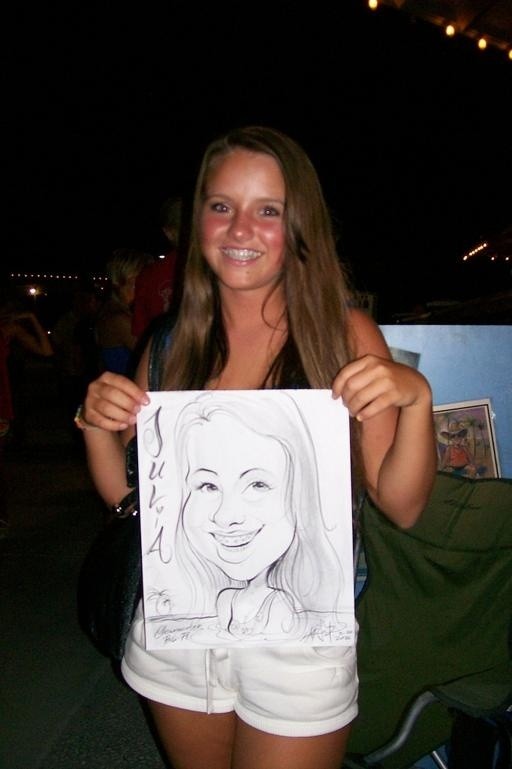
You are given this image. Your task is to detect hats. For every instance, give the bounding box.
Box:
[440,422,468,440]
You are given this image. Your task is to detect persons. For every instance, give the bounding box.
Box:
[441,423,477,479]
[150,392,345,638]
[76,127,439,769]
[3,194,191,445]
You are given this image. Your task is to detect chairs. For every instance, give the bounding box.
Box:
[341,470,512,769]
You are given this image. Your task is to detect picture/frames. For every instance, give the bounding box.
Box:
[432,399,501,480]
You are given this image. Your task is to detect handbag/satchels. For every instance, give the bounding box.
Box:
[75,493,142,685]
